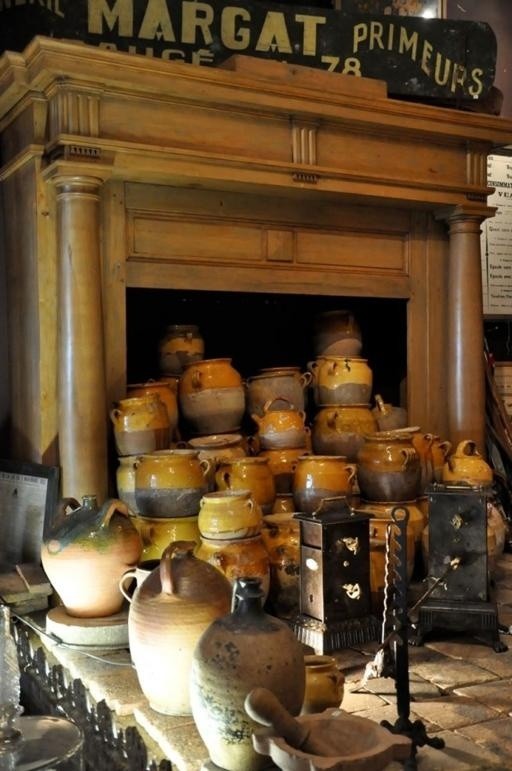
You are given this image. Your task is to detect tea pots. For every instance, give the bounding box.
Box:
[443,440,494,490]
[252,396,306,451]
